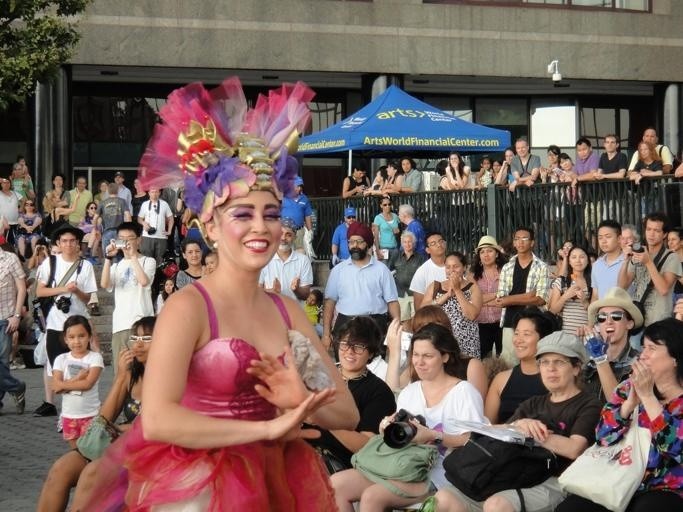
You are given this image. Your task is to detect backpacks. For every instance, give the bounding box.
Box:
[48,253,57,332]
[16,220,41,236]
[442,432,557,501]
[390,270,416,321]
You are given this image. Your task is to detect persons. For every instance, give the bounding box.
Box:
[260,126,682,512]
[116,147,364,512]
[1,157,217,512]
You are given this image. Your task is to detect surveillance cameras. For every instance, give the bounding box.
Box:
[548,61,562,84]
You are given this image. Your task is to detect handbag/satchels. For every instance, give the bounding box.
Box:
[76,414,119,460]
[659,145,681,173]
[350,433,439,498]
[557,404,652,511]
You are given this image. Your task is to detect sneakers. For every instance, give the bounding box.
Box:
[8,359,26,370]
[32,402,57,417]
[10,380,26,414]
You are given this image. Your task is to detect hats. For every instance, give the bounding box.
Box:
[345,222,374,248]
[113,170,124,177]
[293,176,304,186]
[474,234,503,254]
[533,330,587,361]
[51,223,85,246]
[587,287,643,330]
[344,207,356,218]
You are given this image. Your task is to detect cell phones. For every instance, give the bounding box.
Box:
[113,239,128,249]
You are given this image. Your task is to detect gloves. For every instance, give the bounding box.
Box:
[585,334,608,364]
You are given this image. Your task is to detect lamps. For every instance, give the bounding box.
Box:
[548,60,561,81]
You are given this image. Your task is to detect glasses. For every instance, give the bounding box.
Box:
[595,310,629,325]
[336,338,369,354]
[127,335,153,343]
[24,202,35,207]
[89,207,98,210]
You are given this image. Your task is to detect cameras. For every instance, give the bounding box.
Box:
[627,242,644,256]
[148,227,156,235]
[56,296,71,313]
[383,409,429,449]
